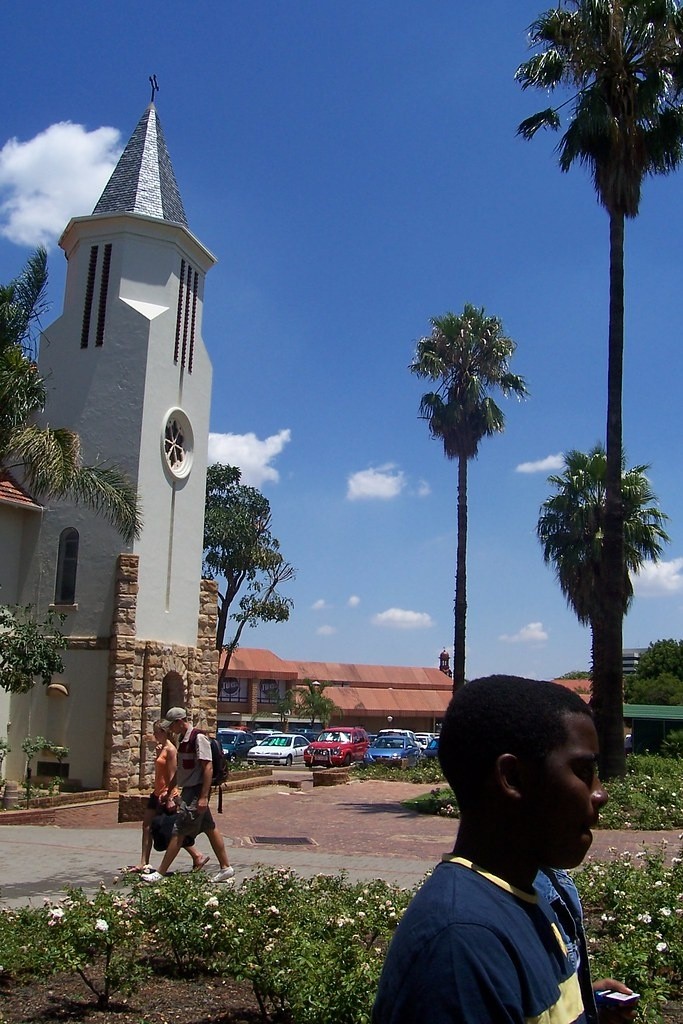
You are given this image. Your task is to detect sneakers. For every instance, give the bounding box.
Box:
[141,871,164,883]
[211,867,235,882]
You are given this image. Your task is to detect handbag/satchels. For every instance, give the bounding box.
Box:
[151,804,194,851]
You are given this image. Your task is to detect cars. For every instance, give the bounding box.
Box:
[230,725,440,759]
[246,733,311,767]
[217,730,259,765]
[363,736,419,767]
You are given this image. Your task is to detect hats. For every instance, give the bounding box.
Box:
[159,707,186,728]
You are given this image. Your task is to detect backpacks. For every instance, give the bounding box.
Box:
[179,729,229,785]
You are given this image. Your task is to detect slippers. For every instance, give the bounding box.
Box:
[192,856,210,873]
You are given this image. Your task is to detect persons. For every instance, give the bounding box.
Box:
[372,674,638,1024]
[139,707,235,884]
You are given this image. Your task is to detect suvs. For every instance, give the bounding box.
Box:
[303,726,371,769]
